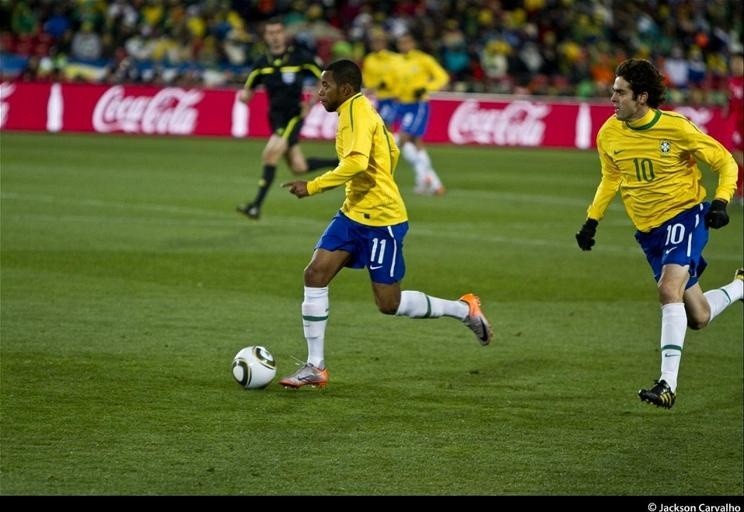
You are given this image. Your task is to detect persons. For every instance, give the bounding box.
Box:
[359,34,451,196]
[575,58,744,409]
[0,0,744,106]
[237,20,341,221]
[279,60,493,388]
[361,36,402,149]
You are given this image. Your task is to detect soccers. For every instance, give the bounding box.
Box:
[232,346,276,389]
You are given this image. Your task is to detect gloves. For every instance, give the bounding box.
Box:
[576,219,598,251]
[705,200,729,229]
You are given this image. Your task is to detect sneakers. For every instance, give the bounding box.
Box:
[279,363,329,388]
[459,293,492,346]
[638,379,676,409]
